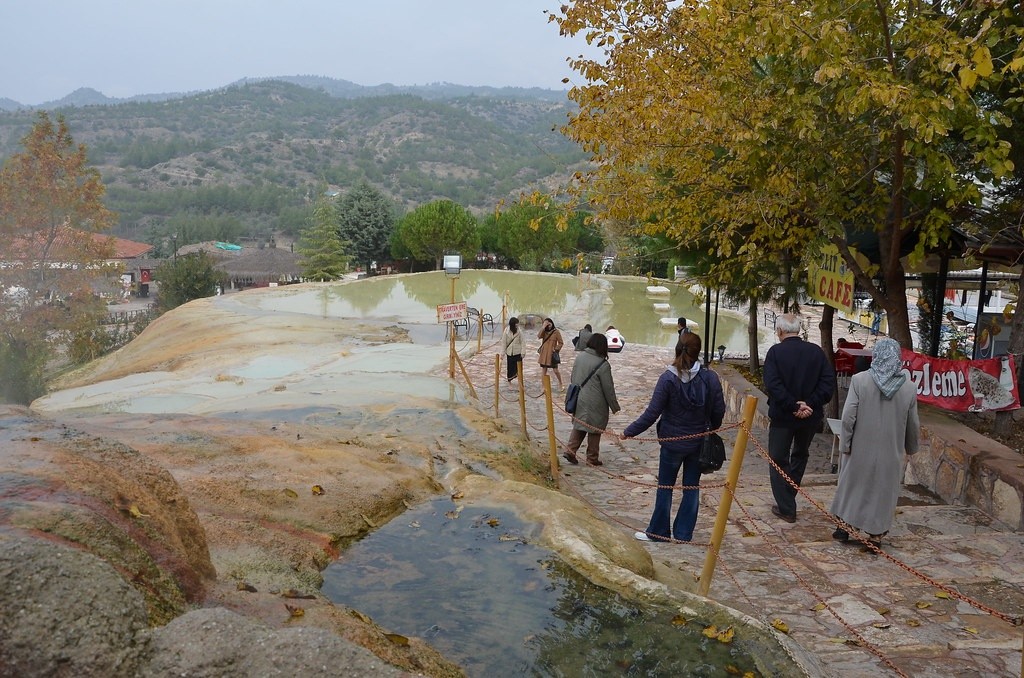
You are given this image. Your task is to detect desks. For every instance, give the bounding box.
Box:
[834,348,872,388]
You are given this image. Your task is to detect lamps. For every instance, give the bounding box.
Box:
[717,345,726,362]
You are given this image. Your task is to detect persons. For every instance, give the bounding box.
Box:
[575,324,593,351]
[563,333,620,466]
[500,317,527,390]
[831,338,919,554]
[619,333,725,542]
[604,325,626,353]
[537,318,564,393]
[677,317,690,341]
[763,314,834,523]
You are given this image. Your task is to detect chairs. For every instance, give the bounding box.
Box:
[444,317,468,341]
[835,357,852,389]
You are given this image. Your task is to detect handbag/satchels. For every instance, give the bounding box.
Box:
[537,349,540,354]
[572,337,580,345]
[698,427,726,474]
[565,384,580,413]
[551,352,560,364]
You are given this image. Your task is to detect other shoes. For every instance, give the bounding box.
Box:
[586,460,602,465]
[563,451,579,464]
[832,528,849,541]
[634,532,653,541]
[866,540,881,555]
[670,532,675,539]
[557,387,564,392]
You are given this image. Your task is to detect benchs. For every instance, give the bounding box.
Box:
[764,308,779,330]
[723,298,739,310]
[465,307,494,339]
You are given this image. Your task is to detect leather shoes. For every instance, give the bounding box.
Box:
[772,506,796,523]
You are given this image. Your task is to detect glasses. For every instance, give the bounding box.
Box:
[774,328,782,335]
